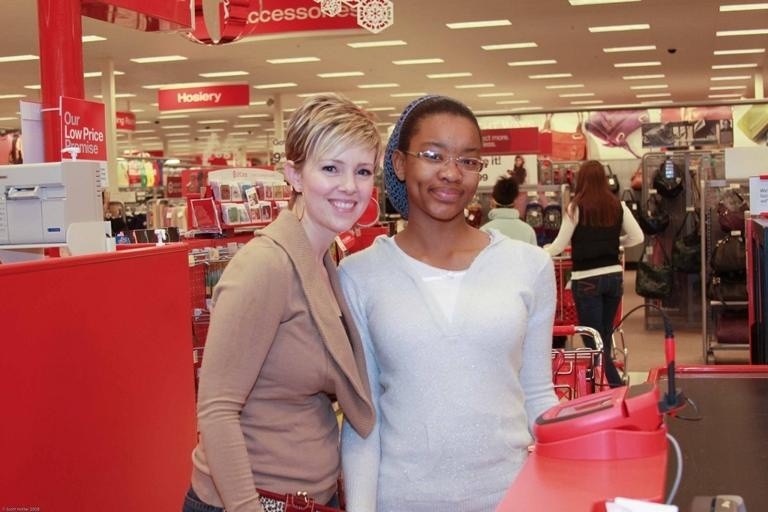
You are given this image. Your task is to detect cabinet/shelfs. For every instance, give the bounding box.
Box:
[107,150,750,366]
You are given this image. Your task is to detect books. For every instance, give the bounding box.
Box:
[210,178,296,225]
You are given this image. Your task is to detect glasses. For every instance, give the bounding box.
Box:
[402,147,486,176]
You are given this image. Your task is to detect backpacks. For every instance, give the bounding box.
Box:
[544,205,562,237]
[524,203,544,233]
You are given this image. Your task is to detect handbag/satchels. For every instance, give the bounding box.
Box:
[652,154,685,198]
[629,161,643,191]
[585,109,652,159]
[671,208,705,274]
[222,487,347,512]
[709,230,758,284]
[634,262,674,299]
[639,196,671,236]
[617,189,641,222]
[540,111,587,161]
[706,276,749,301]
[717,191,750,234]
[601,163,619,194]
[715,309,749,344]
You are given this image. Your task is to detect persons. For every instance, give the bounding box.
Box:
[182,91,383,511]
[476,175,540,246]
[195,92,564,510]
[506,154,527,185]
[543,160,645,389]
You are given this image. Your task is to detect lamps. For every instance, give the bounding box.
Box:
[572,112,583,140]
[540,113,554,134]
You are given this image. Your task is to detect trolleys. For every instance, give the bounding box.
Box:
[552,246,630,401]
